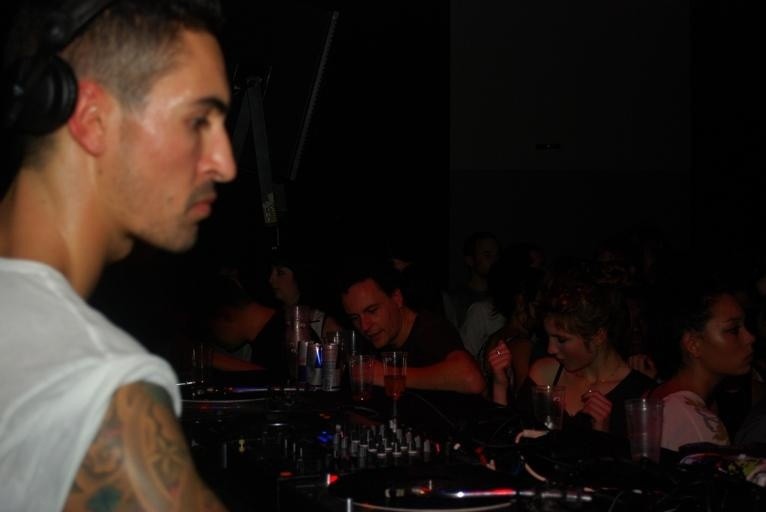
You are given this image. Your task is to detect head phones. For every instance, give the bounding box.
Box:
[0,0,118,137]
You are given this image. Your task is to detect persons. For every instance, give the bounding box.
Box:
[135,263,766,510]
[1,1,237,512]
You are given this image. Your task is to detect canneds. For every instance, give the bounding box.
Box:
[320,343,341,392]
[298,340,314,384]
[305,342,324,388]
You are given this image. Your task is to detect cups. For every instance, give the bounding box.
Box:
[625,397,663,460]
[348,355,375,402]
[531,385,565,431]
[381,351,408,399]
